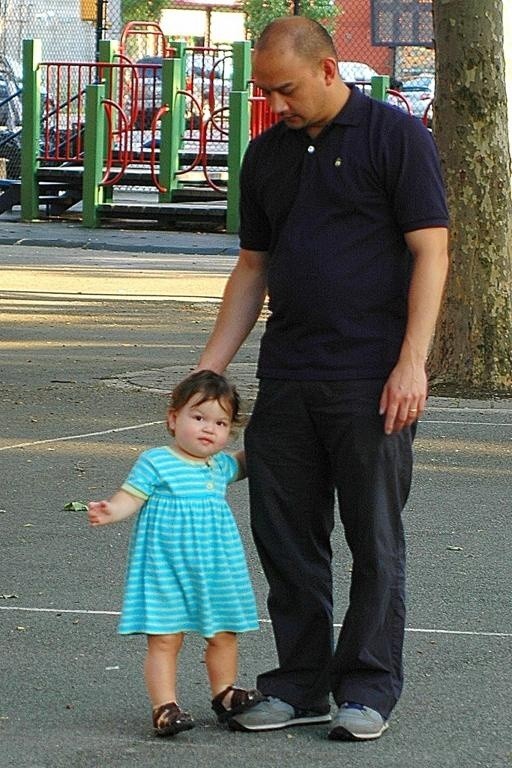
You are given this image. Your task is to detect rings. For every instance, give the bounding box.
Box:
[407,407,418,413]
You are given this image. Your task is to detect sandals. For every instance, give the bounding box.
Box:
[212,686,266,721]
[153,700,195,735]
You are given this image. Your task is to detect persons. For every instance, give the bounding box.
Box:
[166,15,450,741]
[85,368,268,739]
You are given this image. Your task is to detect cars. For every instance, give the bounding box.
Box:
[0,52,61,130]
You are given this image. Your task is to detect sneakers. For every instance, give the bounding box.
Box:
[229,696,332,731]
[329,701,388,740]
